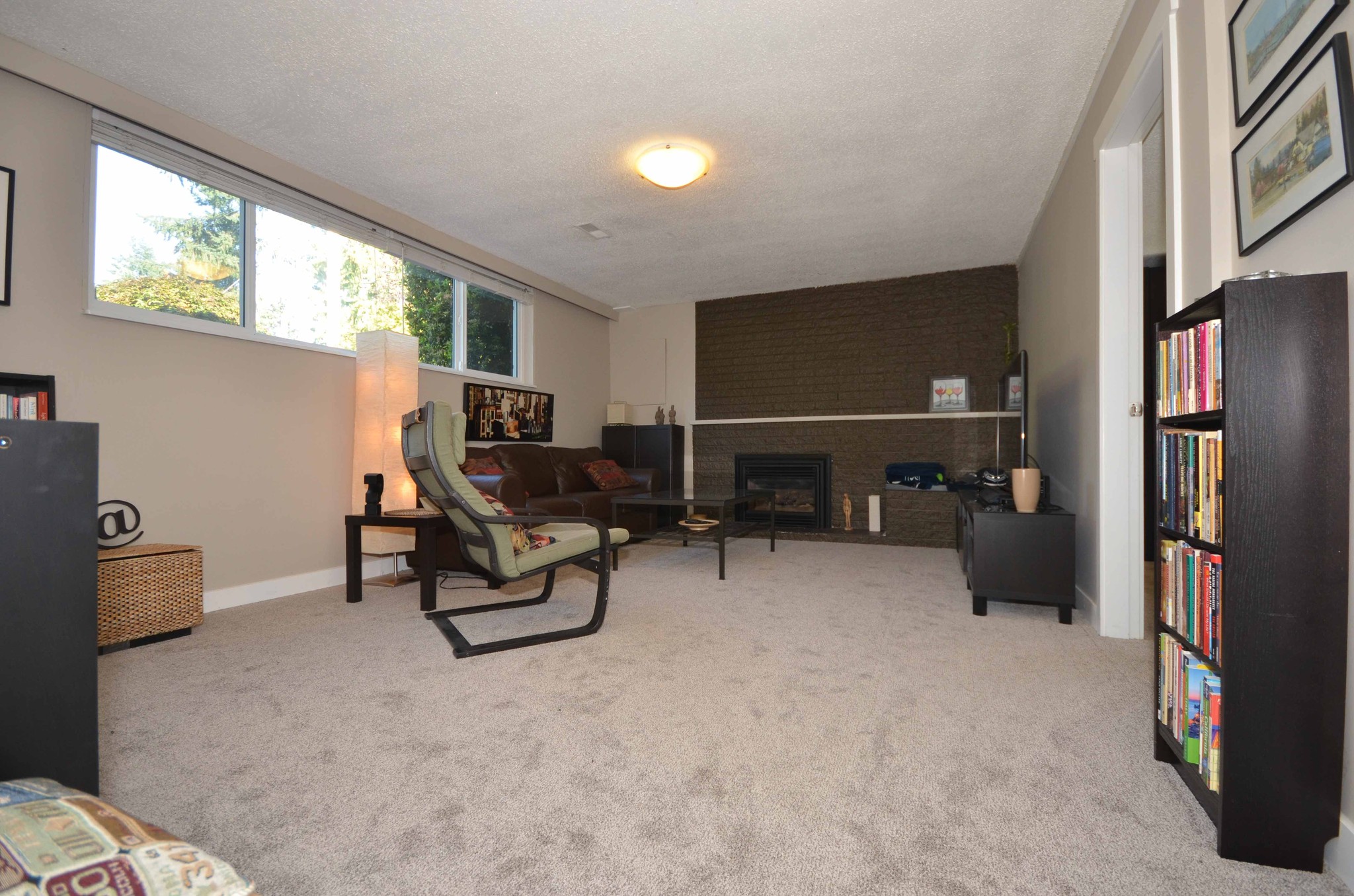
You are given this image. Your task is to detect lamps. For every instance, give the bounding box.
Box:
[352,330,418,588]
[633,144,709,191]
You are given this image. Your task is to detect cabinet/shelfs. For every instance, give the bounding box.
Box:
[955,489,1078,626]
[601,424,685,526]
[1152,272,1348,874]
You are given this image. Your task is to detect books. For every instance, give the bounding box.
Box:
[1159,430,1222,547]
[0,391,48,420]
[1156,319,1222,418]
[1160,540,1222,667]
[1158,633,1221,794]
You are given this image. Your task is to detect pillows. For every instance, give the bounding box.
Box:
[460,458,530,498]
[577,461,638,490]
[0,778,256,896]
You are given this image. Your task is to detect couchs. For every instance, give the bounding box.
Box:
[459,444,661,546]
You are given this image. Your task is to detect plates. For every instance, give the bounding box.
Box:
[679,519,719,530]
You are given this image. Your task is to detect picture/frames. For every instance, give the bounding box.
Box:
[1229,1,1352,125]
[928,374,969,412]
[463,382,554,444]
[0,165,17,308]
[1229,33,1353,256]
[1005,374,1022,411]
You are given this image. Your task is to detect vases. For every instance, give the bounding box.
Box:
[1010,468,1041,512]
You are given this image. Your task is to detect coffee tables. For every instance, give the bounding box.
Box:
[610,490,776,581]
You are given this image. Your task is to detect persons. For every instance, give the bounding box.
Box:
[843,493,852,527]
[669,405,676,424]
[655,406,665,425]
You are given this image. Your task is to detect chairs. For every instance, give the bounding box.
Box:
[399,399,629,659]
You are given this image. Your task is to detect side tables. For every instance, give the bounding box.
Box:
[344,511,455,612]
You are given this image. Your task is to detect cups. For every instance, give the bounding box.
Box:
[1012,468,1040,514]
